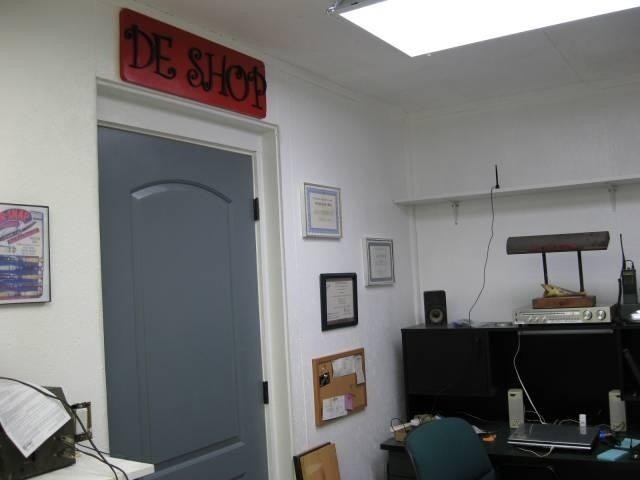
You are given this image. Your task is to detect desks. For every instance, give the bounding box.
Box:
[380,418,640,480]
[27,444,155,480]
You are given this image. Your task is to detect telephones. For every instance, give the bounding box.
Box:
[618,234,640,326]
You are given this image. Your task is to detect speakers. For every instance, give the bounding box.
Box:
[423,289,447,326]
[508,389,526,430]
[608,388,627,433]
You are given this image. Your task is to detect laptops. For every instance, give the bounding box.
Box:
[505,422,599,452]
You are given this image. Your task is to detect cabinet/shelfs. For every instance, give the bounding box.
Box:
[400,319,640,424]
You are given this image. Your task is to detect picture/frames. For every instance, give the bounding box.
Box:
[318,273,358,332]
[362,236,397,287]
[299,180,342,240]
[312,348,367,428]
[0,202,52,306]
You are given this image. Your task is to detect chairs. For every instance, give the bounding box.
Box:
[404,418,496,479]
